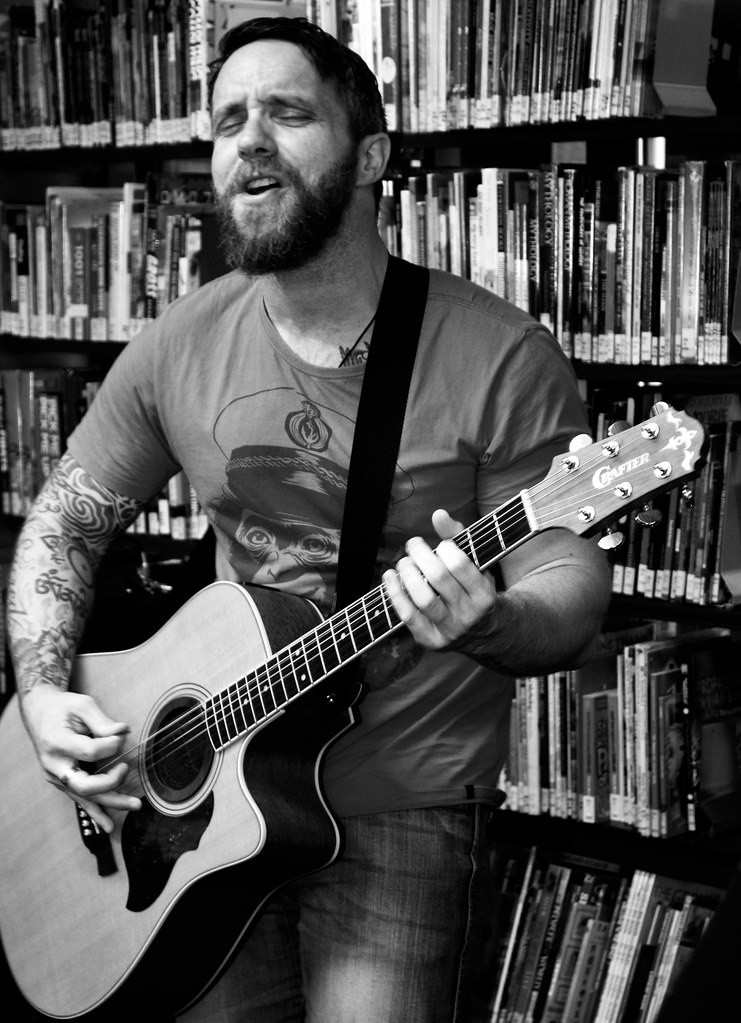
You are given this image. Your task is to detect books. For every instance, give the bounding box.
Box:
[0,1,741,1023]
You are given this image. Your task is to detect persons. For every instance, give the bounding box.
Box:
[6,15,612,1023]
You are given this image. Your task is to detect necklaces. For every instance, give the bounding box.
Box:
[338,315,375,368]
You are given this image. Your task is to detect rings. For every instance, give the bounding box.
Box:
[62,767,81,786]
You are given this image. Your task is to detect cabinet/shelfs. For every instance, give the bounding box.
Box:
[0,1,741,1023]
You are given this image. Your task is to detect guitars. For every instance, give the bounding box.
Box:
[0,396,709,1022]
